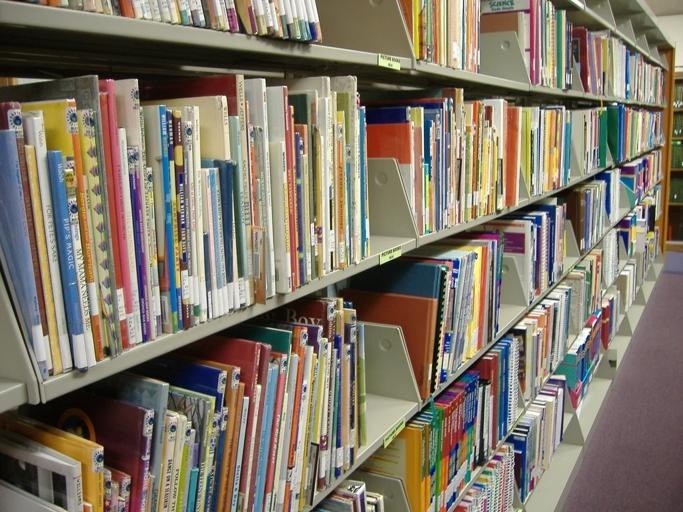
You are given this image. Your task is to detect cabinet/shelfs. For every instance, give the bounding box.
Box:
[663,72,683,253]
[0,0,676,512]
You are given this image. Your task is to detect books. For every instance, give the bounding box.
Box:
[1,2,670,512]
